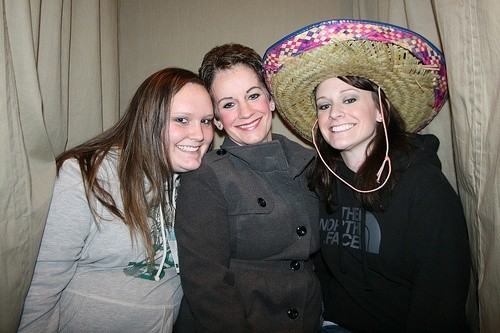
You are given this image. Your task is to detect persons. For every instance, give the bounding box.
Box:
[260,19,472,332]
[169,43,324,333]
[17,66,215,333]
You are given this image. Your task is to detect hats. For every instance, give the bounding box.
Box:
[262,20,449,147]
